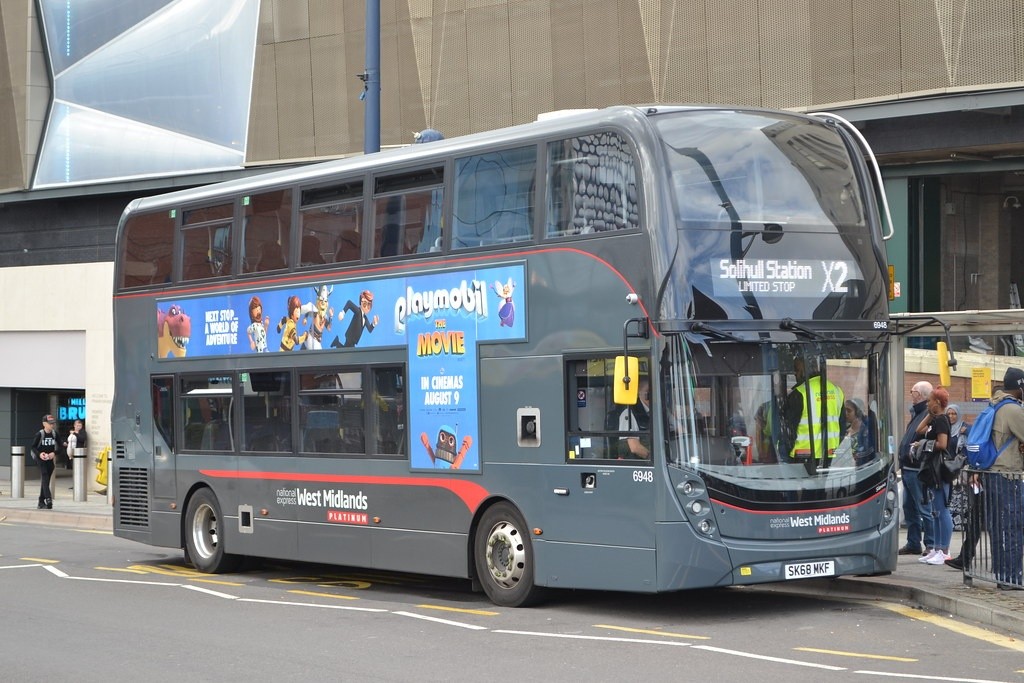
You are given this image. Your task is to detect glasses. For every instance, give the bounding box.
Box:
[909,389,919,394]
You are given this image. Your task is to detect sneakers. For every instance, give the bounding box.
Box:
[926,549,951,565]
[945,553,972,571]
[918,549,939,563]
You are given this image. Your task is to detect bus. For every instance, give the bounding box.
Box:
[110,102,958,610]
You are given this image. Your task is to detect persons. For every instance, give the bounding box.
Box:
[755,354,868,464]
[62,420,86,490]
[617,380,687,459]
[32,415,63,508]
[898,367,1024,590]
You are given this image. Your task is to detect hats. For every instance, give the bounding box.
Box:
[1003,367,1024,390]
[41,414,57,424]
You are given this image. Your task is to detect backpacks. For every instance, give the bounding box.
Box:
[30,429,56,461]
[965,398,1022,470]
[939,448,968,483]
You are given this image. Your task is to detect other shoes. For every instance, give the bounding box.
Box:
[922,547,934,555]
[997,577,1024,590]
[899,541,922,554]
[38,497,52,509]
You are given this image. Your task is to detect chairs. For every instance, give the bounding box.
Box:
[186,411,348,453]
[183,223,442,280]
